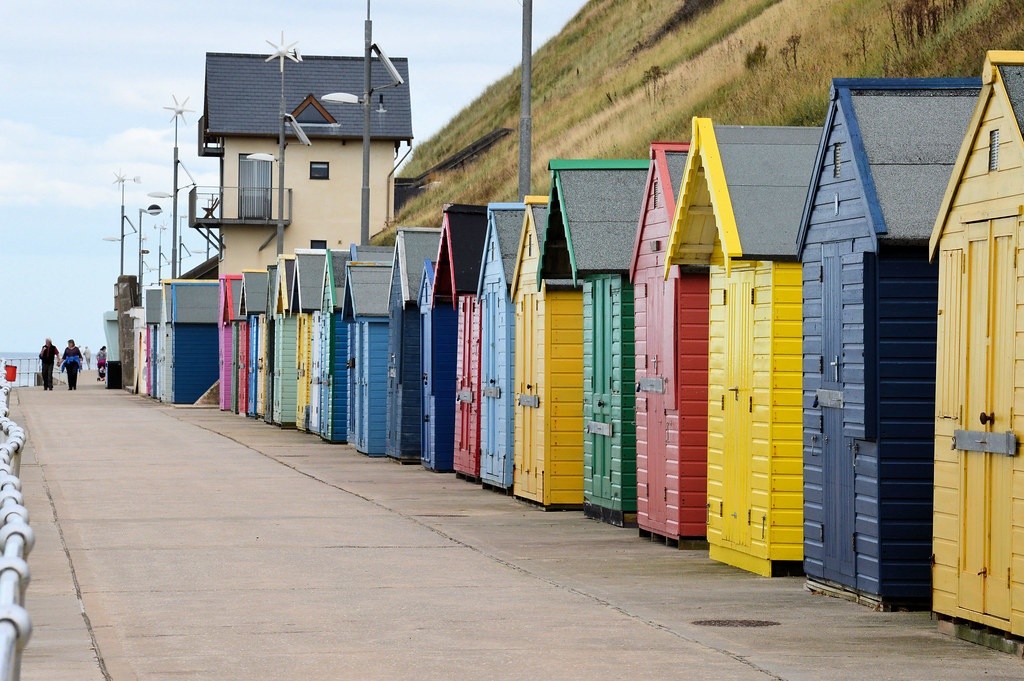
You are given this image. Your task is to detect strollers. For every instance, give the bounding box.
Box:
[98,358,106,381]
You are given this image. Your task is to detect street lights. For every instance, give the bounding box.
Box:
[320,91,370,246]
[138,204,162,308]
[247,153,285,256]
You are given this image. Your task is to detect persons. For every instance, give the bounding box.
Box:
[95,346,106,362]
[38,338,61,392]
[56,340,82,391]
[82,347,91,371]
[98,366,105,381]
